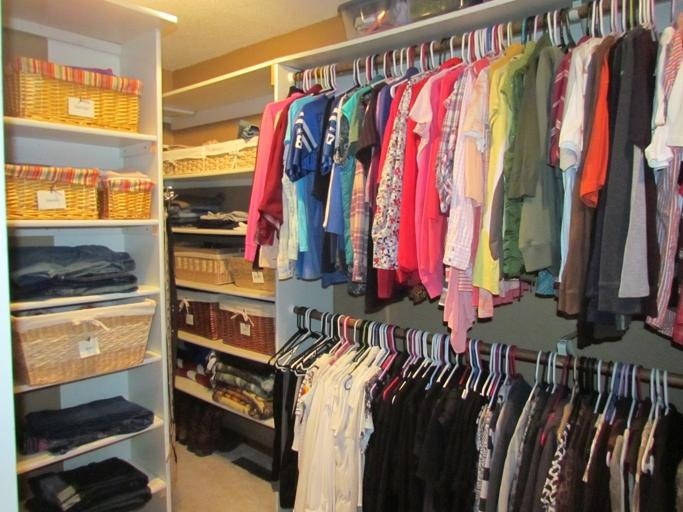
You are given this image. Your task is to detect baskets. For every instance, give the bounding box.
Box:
[173,247,244,285]
[5,163,101,220]
[164,151,174,176]
[204,139,235,173]
[228,256,277,291]
[219,296,276,356]
[97,177,156,220]
[176,289,227,341]
[11,297,156,386]
[235,136,259,169]
[3,56,143,133]
[174,146,205,176]
